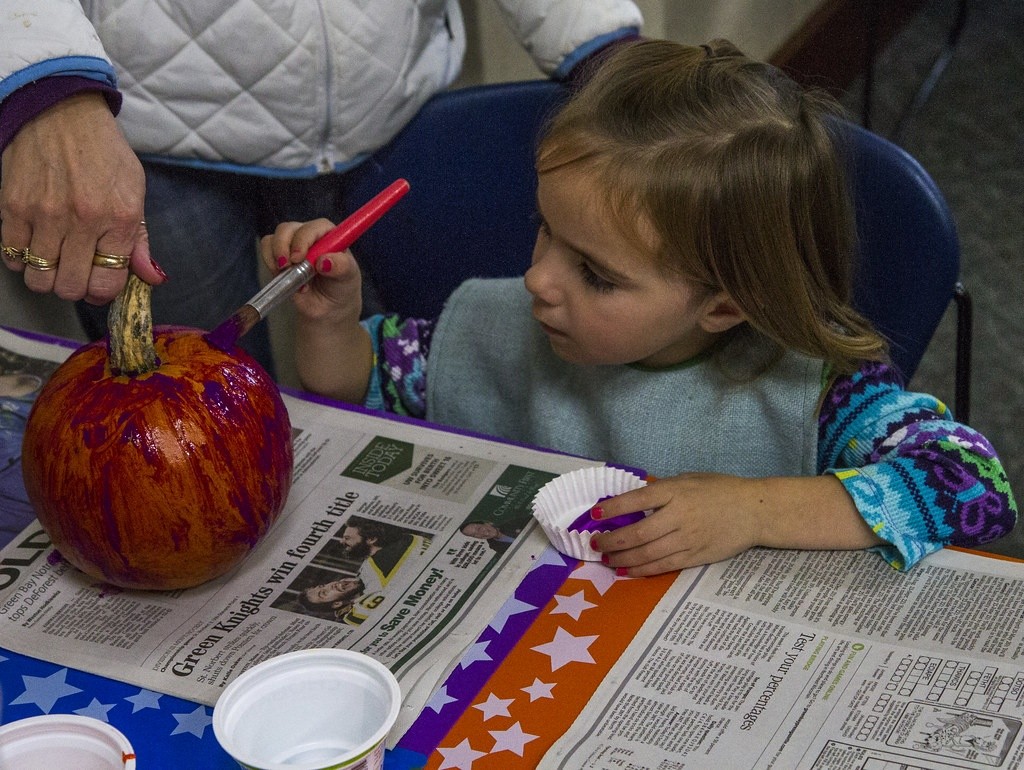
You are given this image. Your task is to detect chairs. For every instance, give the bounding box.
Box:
[350,62,982,422]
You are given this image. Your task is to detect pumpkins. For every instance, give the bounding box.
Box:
[23,269,297,590]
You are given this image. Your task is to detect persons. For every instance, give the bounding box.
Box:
[260,35,1020,580]
[2,0,653,363]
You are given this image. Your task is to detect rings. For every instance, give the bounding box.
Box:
[26,254,60,274]
[91,250,134,270]
[0,242,29,259]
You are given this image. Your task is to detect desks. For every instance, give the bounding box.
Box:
[1,322,1024,769]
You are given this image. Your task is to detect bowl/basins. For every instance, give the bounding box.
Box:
[0,714,136,770]
[211,647,402,770]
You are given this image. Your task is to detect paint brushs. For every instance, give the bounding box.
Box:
[198,176,415,355]
